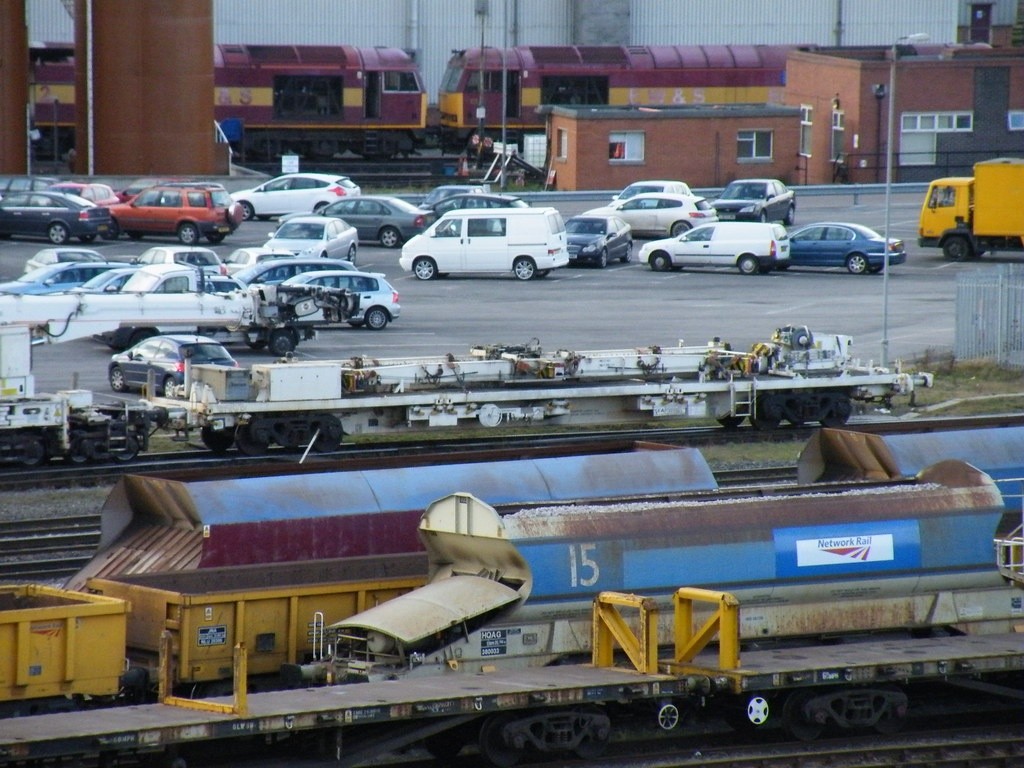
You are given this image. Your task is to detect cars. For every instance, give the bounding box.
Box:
[230,173,361,222]
[710,179,796,227]
[49,183,120,207]
[262,217,357,266]
[0,192,111,244]
[0,248,400,398]
[278,196,436,248]
[416,185,528,218]
[773,222,906,275]
[0,175,57,198]
[565,182,790,275]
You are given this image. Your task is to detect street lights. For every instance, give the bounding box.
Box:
[880,32,929,367]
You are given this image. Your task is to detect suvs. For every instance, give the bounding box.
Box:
[99,182,243,246]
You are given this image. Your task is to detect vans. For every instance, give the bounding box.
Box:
[398,208,571,282]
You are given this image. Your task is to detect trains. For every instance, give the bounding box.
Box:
[62,415,1024,586]
[0,588,1024,768]
[27,42,804,163]
[0,285,935,467]
[279,461,1024,690]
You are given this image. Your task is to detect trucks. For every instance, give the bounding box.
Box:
[918,161,1024,261]
